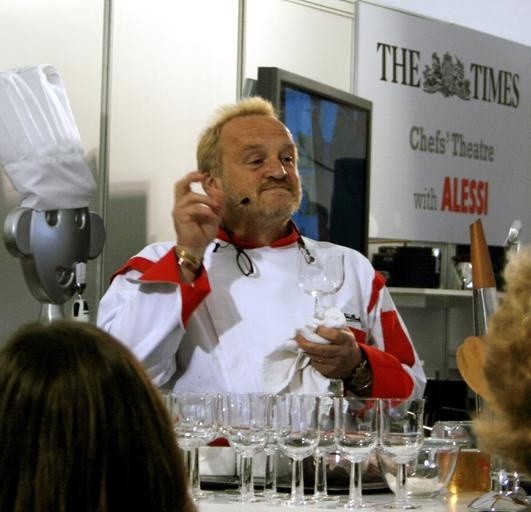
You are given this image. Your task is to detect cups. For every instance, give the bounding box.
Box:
[437,420,492,495]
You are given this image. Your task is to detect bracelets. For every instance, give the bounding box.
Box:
[175,246,202,276]
[343,359,373,396]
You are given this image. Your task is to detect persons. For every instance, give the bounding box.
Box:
[96,98,427,479]
[0,320,197,512]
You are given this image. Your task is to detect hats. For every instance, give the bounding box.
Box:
[0,51,96,210]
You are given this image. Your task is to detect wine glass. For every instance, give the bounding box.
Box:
[158,391,426,512]
[298,250,345,318]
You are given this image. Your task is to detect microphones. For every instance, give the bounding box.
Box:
[217,194,252,210]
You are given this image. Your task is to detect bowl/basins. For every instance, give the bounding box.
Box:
[369,437,467,499]
